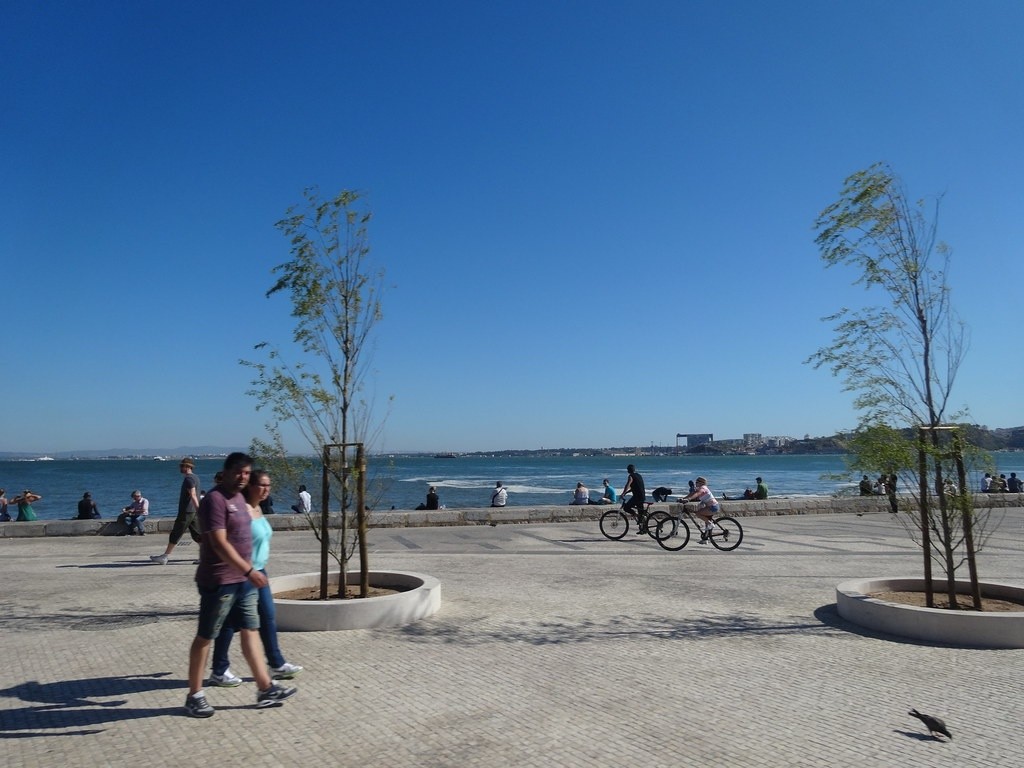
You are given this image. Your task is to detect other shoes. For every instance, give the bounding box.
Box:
[636,530,647,534]
[704,523,716,531]
[697,539,708,545]
[636,514,644,525]
[140,532,145,535]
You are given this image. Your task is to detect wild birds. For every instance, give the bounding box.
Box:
[909,708,953,740]
[365,505,369,510]
[391,505,395,511]
[857,512,864,517]
[489,520,497,527]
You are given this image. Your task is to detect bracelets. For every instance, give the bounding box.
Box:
[243,567,254,576]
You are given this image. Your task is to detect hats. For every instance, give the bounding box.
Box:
[180,458,195,468]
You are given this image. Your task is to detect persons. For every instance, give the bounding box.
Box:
[860,470,899,514]
[291,485,312,513]
[653,487,672,502]
[743,477,768,500]
[185,452,298,718]
[569,482,589,505]
[944,473,1024,497]
[426,486,439,510]
[678,476,719,545]
[150,458,202,565]
[0,488,13,522]
[200,472,276,515]
[78,492,101,519]
[490,481,508,507]
[120,490,150,535]
[598,478,616,504]
[208,469,303,687]
[619,464,647,535]
[9,490,42,521]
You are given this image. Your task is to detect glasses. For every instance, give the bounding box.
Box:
[256,483,272,488]
[696,482,699,483]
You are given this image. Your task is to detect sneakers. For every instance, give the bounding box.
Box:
[150,555,169,565]
[257,679,298,707]
[184,690,215,718]
[208,667,243,687]
[193,559,201,564]
[268,663,304,678]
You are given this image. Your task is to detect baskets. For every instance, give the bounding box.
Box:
[669,503,684,516]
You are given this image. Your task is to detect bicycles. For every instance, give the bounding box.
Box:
[599,494,676,541]
[655,498,743,551]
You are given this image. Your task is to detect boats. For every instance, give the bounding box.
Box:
[153,457,166,461]
[35,455,55,464]
[434,453,456,458]
